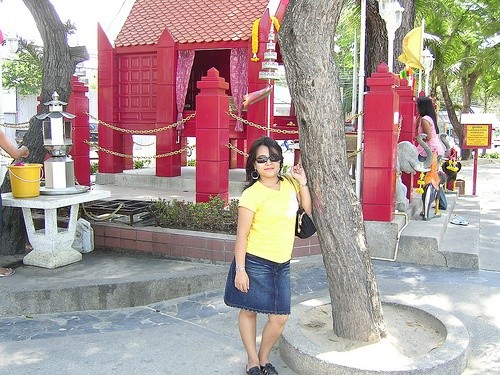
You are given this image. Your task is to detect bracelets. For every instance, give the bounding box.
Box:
[298,183,308,187]
[236,266,245,272]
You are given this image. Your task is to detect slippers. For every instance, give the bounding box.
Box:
[245,365,263,375]
[260,363,278,375]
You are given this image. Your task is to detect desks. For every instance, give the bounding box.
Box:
[1,190,111,268]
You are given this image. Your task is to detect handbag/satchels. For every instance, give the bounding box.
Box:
[281,173,317,239]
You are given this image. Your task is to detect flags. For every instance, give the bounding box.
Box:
[397,19,425,71]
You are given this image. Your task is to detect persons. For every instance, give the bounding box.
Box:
[416,97,447,210]
[224,138,312,375]
[0,131,29,276]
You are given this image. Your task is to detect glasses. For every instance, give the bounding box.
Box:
[254,154,281,163]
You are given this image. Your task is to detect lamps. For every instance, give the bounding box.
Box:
[35,91,90,194]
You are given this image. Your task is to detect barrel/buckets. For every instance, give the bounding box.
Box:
[6,163,43,197]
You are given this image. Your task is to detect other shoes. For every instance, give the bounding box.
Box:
[451,214,469,226]
[0,267,15,277]
